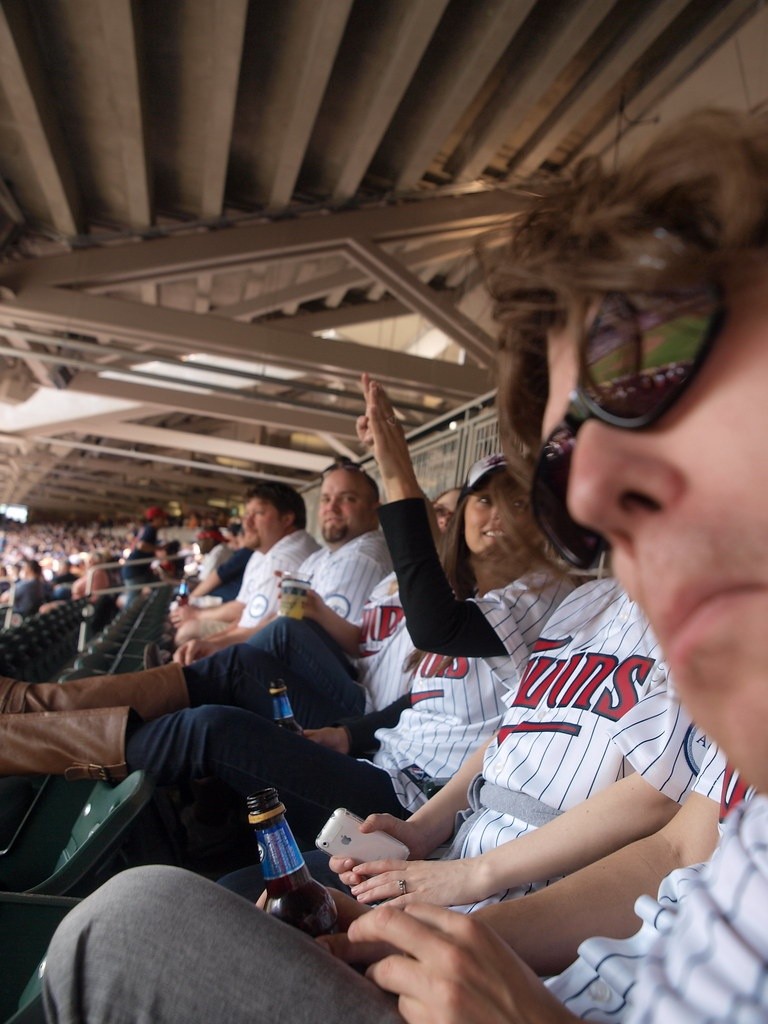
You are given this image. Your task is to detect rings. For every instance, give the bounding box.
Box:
[387,414,397,427]
[398,880,407,896]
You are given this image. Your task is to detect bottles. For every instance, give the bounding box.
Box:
[176,579,188,606]
[246,788,340,938]
[268,679,303,733]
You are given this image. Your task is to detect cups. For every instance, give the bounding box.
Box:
[278,571,312,620]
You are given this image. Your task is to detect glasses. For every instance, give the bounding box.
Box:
[528,228,724,568]
[322,461,376,492]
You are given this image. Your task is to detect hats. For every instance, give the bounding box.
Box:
[455,453,507,512]
[145,507,169,522]
[198,529,231,542]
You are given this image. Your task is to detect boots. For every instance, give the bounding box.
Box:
[0,663,191,720]
[0,704,146,781]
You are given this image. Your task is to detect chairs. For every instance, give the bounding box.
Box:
[0,769,155,895]
[0,586,175,684]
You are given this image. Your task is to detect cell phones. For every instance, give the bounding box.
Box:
[316,807,409,877]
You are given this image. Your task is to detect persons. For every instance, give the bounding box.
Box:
[198,575,718,948]
[489,94,768,1024]
[1,375,588,875]
[38,734,761,1024]
